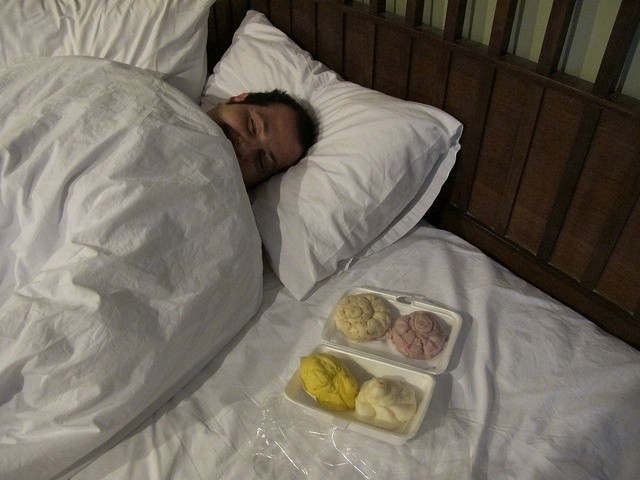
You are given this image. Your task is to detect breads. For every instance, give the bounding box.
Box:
[334,291,391,343]
[355,376,417,427]
[389,310,446,360]
[299,353,357,410]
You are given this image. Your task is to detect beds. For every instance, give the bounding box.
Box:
[0,2,639,479]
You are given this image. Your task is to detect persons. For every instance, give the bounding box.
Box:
[202,88,318,192]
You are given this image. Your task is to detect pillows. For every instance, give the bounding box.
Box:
[1,1,215,104]
[195,7,466,302]
